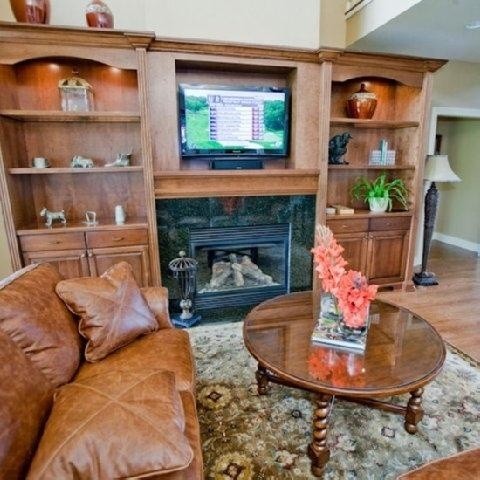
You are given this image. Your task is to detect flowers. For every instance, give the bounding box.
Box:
[311,223,378,329]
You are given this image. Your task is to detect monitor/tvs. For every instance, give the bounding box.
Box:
[177,82,291,170]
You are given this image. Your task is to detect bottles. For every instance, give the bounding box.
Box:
[114,204,126,224]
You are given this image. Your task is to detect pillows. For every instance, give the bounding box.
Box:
[23,369,195,480]
[54,261,159,362]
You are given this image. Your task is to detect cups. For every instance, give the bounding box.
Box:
[31,158,52,169]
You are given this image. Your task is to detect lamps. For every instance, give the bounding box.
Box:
[413,151,461,286]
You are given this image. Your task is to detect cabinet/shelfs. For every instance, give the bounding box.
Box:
[314,44,449,295]
[0,20,164,289]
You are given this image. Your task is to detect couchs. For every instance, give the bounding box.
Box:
[0,258,205,480]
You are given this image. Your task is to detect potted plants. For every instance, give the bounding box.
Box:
[350,170,411,214]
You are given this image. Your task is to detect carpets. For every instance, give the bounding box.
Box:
[171,316,480,480]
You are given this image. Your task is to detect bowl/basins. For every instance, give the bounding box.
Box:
[345,98,378,119]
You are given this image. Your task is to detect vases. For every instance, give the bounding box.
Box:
[335,317,369,341]
[10,0,114,29]
